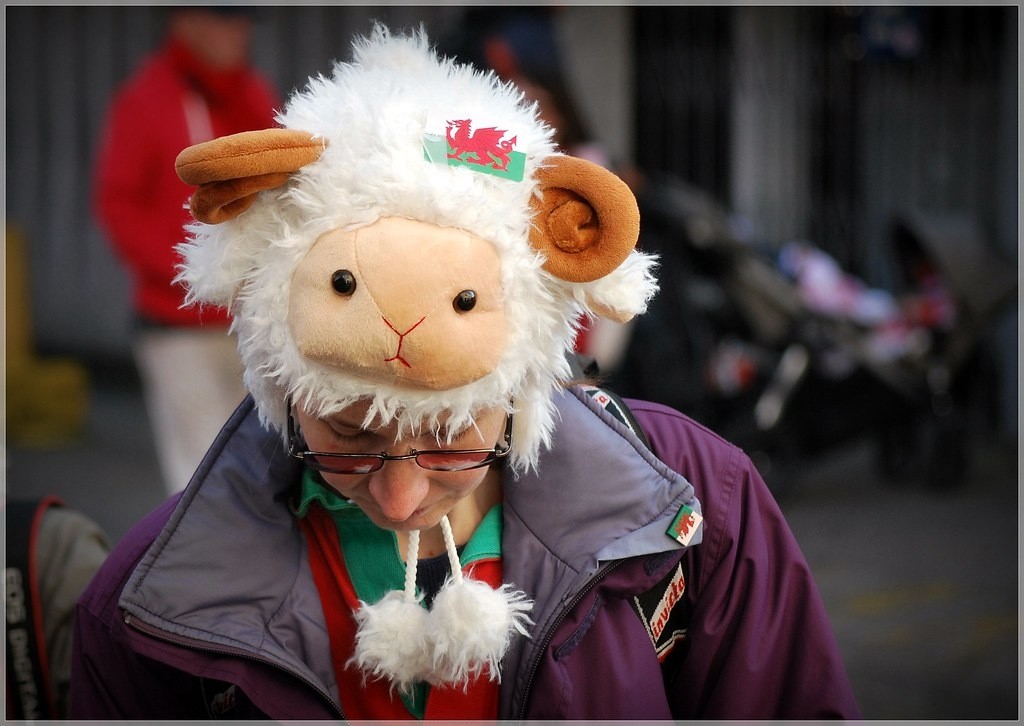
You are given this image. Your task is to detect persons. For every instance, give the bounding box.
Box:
[88,5,969,499]
[63,19,866,721]
[6,492,115,720]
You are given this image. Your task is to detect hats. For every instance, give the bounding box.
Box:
[172,23,655,696]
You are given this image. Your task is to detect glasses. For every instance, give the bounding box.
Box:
[286,393,517,475]
